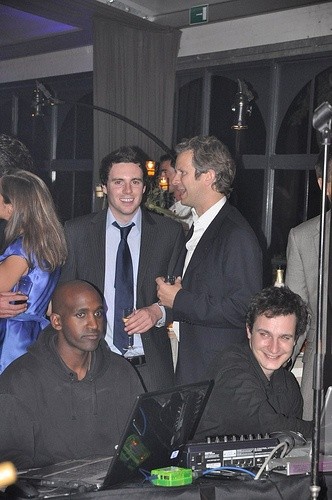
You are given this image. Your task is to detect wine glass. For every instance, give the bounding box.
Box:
[122,308,135,350]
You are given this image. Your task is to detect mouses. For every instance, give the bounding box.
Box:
[5,479,40,498]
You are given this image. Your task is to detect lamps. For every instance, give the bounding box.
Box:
[31,81,55,117]
[230,77,254,130]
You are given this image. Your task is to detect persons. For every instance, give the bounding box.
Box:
[156,135,264,388]
[0,130,71,375]
[0,279,149,496]
[183,287,315,442]
[285,145,332,436]
[63,145,176,393]
[158,151,192,226]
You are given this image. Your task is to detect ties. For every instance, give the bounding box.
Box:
[111,221,136,355]
[172,221,195,279]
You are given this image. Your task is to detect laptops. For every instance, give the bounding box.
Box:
[14,379,215,491]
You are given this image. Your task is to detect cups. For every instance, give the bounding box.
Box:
[162,274,176,285]
[14,281,33,306]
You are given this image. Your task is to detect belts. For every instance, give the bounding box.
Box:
[128,355,144,365]
